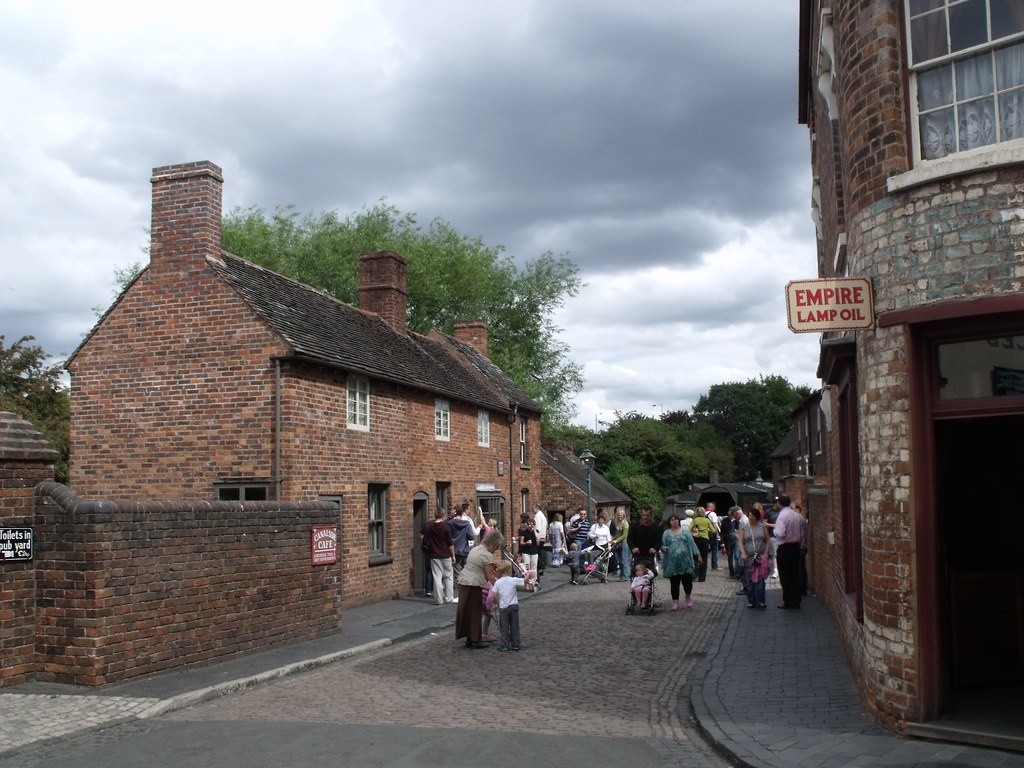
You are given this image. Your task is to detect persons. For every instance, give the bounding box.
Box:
[629,506,661,608]
[548,508,628,584]
[791,503,808,597]
[764,497,780,578]
[533,502,547,575]
[630,564,654,609]
[661,514,702,609]
[481,562,500,641]
[738,509,770,608]
[521,519,540,583]
[752,501,764,523]
[774,492,804,610]
[681,502,749,596]
[518,513,529,556]
[493,561,532,651]
[456,530,504,648]
[513,555,527,577]
[419,503,499,604]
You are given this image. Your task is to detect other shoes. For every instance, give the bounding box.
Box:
[747,603,755,607]
[737,590,745,595]
[641,603,645,608]
[777,603,800,609]
[510,645,519,650]
[636,602,641,607]
[617,579,627,582]
[497,646,508,651]
[472,642,490,648]
[466,641,472,647]
[671,603,679,611]
[685,596,692,608]
[482,634,498,641]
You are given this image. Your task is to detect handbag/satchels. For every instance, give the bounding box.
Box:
[692,526,699,537]
[751,553,762,567]
[560,543,568,555]
[581,539,592,550]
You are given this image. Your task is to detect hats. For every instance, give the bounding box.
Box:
[729,506,741,517]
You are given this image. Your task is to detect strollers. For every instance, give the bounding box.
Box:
[624,552,660,617]
[574,540,616,586]
[501,545,541,593]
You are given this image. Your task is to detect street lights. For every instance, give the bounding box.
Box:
[579,442,596,527]
